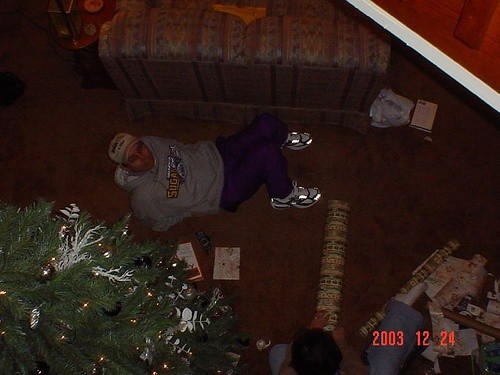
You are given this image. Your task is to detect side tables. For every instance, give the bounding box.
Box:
[48,0,117,50]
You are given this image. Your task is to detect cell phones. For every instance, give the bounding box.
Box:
[194,230,212,253]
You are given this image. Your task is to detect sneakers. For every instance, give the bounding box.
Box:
[270,180,320,209]
[281,131,313,151]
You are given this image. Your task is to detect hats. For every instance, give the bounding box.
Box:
[107,133,140,165]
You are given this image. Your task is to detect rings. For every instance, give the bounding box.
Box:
[314,317,318,320]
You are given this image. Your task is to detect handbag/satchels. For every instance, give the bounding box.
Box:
[0,70,25,105]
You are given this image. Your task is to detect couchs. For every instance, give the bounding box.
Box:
[98,0,391,137]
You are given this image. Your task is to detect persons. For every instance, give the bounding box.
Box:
[108,111,322,232]
[268,280,427,375]
